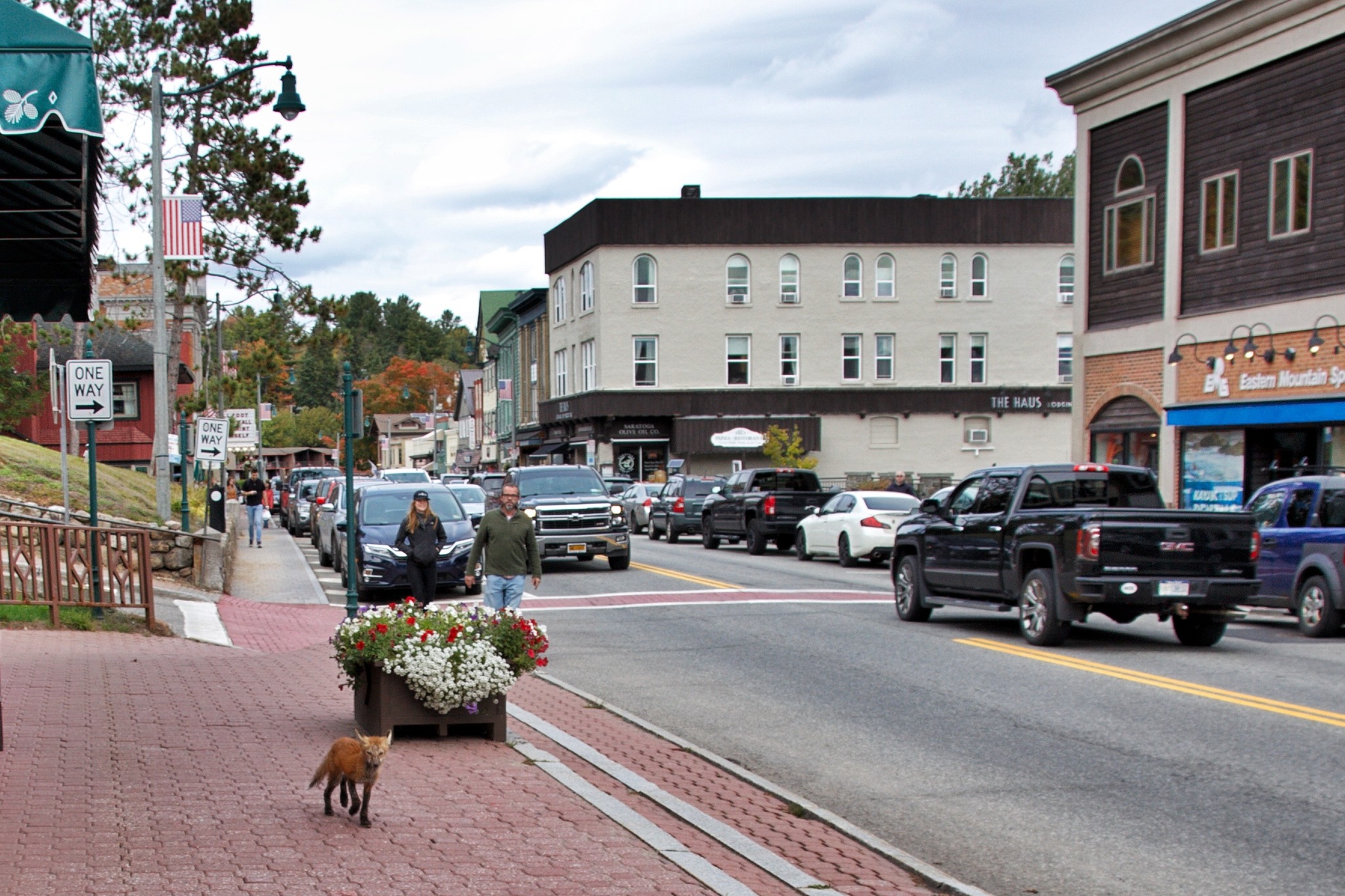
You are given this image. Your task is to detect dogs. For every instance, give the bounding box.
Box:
[306,729,392,829]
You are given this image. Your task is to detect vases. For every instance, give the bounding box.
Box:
[349,665,508,745]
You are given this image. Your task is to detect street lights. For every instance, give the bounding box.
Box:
[255,367,299,483]
[401,384,439,480]
[364,415,394,470]
[214,285,287,496]
[465,335,520,468]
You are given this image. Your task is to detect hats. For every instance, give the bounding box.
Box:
[414,490,431,500]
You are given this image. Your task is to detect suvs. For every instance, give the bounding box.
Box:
[648,474,731,544]
[501,464,632,570]
[276,468,344,527]
[467,472,510,497]
[372,468,471,486]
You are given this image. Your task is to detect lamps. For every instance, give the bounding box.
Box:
[765,412,772,418]
[810,411,817,417]
[1168,333,1214,371]
[1223,322,1298,365]
[1309,313,1345,356]
[716,412,723,417]
[997,409,1005,417]
[859,412,866,418]
[953,410,961,418]
[1043,409,1050,417]
[903,410,911,418]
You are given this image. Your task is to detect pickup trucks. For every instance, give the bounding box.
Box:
[701,467,845,554]
[889,461,1264,647]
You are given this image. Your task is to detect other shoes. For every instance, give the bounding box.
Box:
[256,540,263,547]
[249,540,253,546]
[263,523,268,528]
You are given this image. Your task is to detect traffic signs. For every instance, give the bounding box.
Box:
[63,360,117,423]
[194,418,229,463]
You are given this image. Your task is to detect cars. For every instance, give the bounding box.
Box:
[926,486,979,511]
[269,476,288,507]
[1235,475,1345,638]
[602,478,639,495]
[317,480,401,572]
[622,482,667,533]
[305,478,347,546]
[795,490,923,568]
[338,482,486,601]
[446,484,488,528]
[288,479,320,537]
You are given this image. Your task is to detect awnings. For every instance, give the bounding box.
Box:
[0,0,107,327]
[422,429,445,440]
[421,460,434,471]
[528,442,566,460]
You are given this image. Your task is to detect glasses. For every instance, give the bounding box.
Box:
[502,494,519,498]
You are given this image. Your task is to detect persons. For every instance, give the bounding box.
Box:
[886,471,921,499]
[464,485,541,612]
[227,468,273,548]
[395,491,447,612]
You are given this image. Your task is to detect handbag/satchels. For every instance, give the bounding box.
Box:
[263,508,271,520]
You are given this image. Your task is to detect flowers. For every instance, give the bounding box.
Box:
[329,597,548,714]
